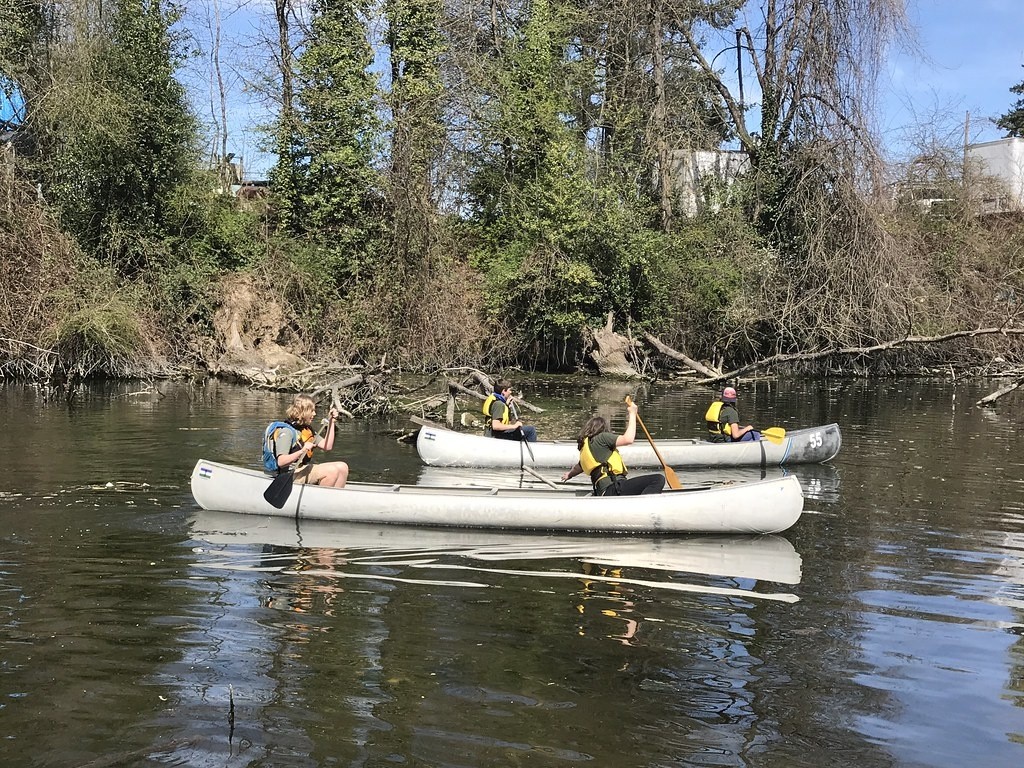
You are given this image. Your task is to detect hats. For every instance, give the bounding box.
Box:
[722,387,736,402]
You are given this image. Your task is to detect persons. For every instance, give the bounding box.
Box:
[485,379,537,442]
[273,394,349,489]
[560,401,666,496]
[708,387,760,443]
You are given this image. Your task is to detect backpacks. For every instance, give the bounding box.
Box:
[261,420,296,475]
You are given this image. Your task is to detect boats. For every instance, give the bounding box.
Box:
[416,420,843,469]
[188,459,805,536]
[189,510,805,587]
[414,467,841,506]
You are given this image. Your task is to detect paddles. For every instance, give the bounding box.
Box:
[624,395,682,489]
[509,401,535,463]
[738,425,786,445]
[263,407,340,510]
